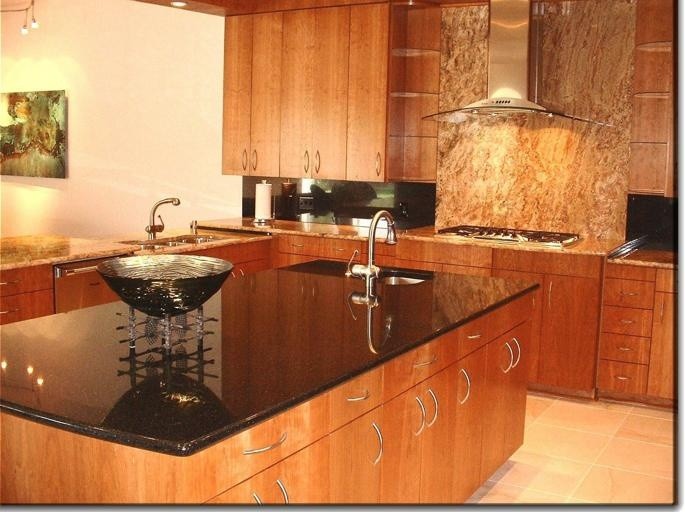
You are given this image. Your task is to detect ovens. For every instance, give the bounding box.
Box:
[55,255,127,314]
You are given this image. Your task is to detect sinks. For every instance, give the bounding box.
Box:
[372,267,432,285]
[113,240,193,252]
[161,234,242,244]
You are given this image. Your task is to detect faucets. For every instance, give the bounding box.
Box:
[345,208,398,279]
[144,198,180,240]
[347,279,393,354]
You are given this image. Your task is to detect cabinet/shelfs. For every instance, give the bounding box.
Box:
[347,1,385,183]
[594,257,672,400]
[277,234,362,267]
[280,4,350,182]
[183,294,526,501]
[222,11,281,179]
[1,264,55,322]
[492,268,592,392]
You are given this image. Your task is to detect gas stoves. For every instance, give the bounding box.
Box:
[434,225,583,250]
